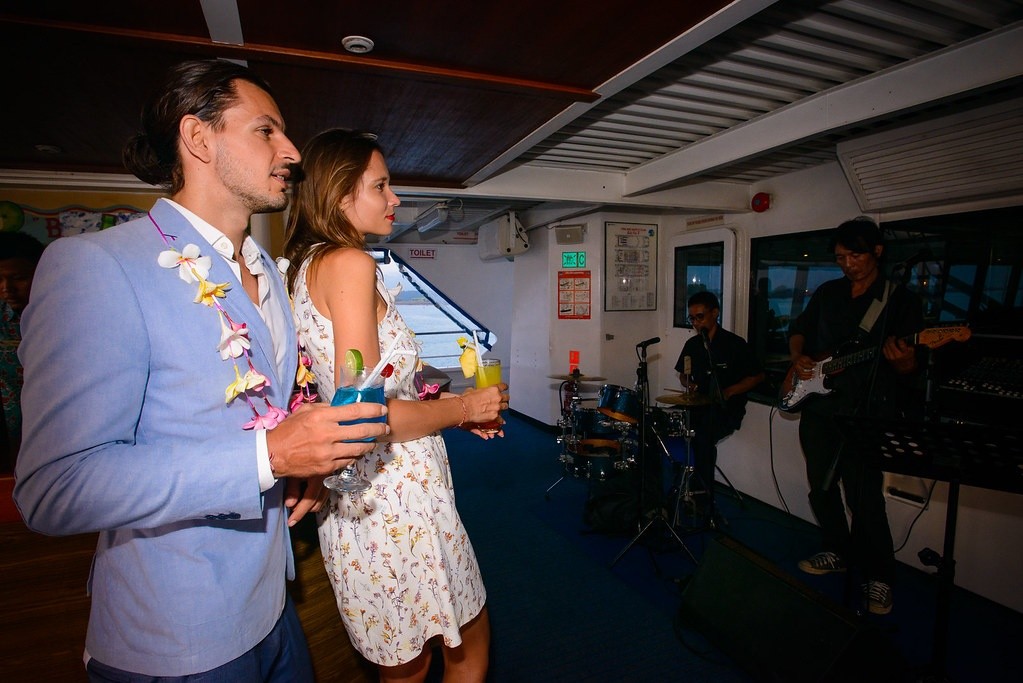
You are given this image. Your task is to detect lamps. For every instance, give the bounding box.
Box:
[414,205,448,232]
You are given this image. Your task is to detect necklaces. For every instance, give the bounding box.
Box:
[146,204,317,445]
[380,273,441,400]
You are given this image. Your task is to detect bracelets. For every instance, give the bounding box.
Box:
[454,393,467,429]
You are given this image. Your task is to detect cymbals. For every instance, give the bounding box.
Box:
[655,394,712,405]
[544,373,608,381]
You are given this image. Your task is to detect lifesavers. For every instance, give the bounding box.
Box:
[596,383,642,422]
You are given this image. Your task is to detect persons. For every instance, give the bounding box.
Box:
[275,131,491,683]
[673,291,764,521]
[12,59,306,683]
[786,215,917,615]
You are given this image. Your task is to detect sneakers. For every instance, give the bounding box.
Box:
[798,552,847,575]
[861,577,892,615]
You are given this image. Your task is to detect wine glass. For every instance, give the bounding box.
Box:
[322,364,388,494]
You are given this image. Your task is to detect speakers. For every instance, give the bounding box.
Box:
[555,224,584,245]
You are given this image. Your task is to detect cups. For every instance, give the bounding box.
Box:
[475,358,503,433]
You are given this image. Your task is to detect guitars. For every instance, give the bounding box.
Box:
[774,323,970,409]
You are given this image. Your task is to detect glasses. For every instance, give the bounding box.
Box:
[687,311,711,322]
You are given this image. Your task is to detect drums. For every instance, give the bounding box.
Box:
[566,435,638,482]
[569,399,634,440]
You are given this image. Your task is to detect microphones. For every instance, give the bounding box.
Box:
[701,327,711,349]
[637,336,661,347]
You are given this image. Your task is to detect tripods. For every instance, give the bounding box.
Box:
[606,346,730,571]
